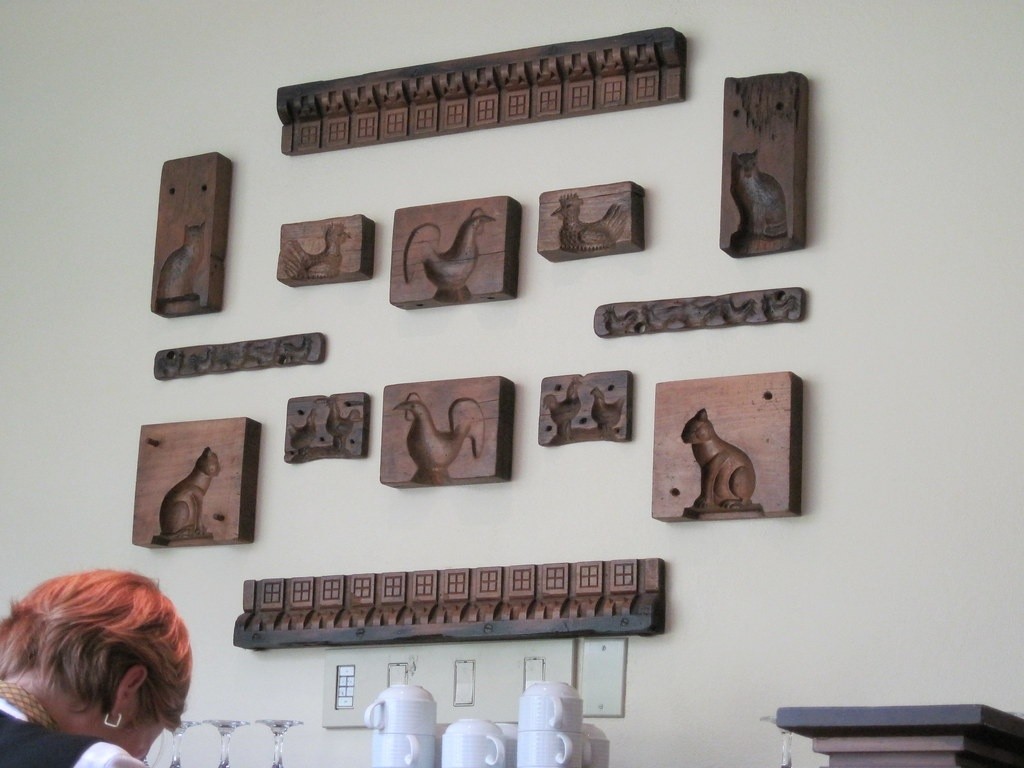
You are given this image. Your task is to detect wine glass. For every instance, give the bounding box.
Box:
[256,719,303,768]
[203,720,250,768]
[166,721,201,768]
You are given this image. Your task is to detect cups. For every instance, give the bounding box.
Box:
[495,723,518,768]
[364,685,437,735]
[369,734,435,768]
[441,719,506,768]
[516,732,581,768]
[580,724,610,767]
[516,681,584,732]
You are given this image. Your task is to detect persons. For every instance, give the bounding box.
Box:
[0,570,192,768]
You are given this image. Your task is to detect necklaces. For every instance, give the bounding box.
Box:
[0,681,58,730]
